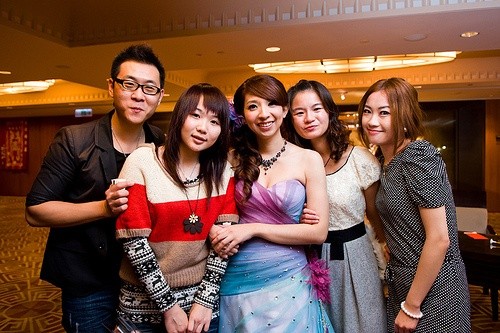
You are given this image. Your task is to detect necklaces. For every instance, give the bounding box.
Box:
[183,179,204,234]
[177,160,198,180]
[111,128,141,158]
[324,157,330,167]
[258,139,287,176]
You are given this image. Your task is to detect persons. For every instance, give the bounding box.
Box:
[25,45,470,333]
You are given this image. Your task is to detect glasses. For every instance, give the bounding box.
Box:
[112,76,161,95]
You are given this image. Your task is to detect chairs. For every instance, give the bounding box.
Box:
[455,206,495,233]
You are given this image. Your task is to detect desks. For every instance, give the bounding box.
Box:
[457,230,500,320]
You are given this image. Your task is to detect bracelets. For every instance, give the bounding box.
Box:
[400,301,423,319]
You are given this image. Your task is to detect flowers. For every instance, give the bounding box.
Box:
[228,99,247,134]
